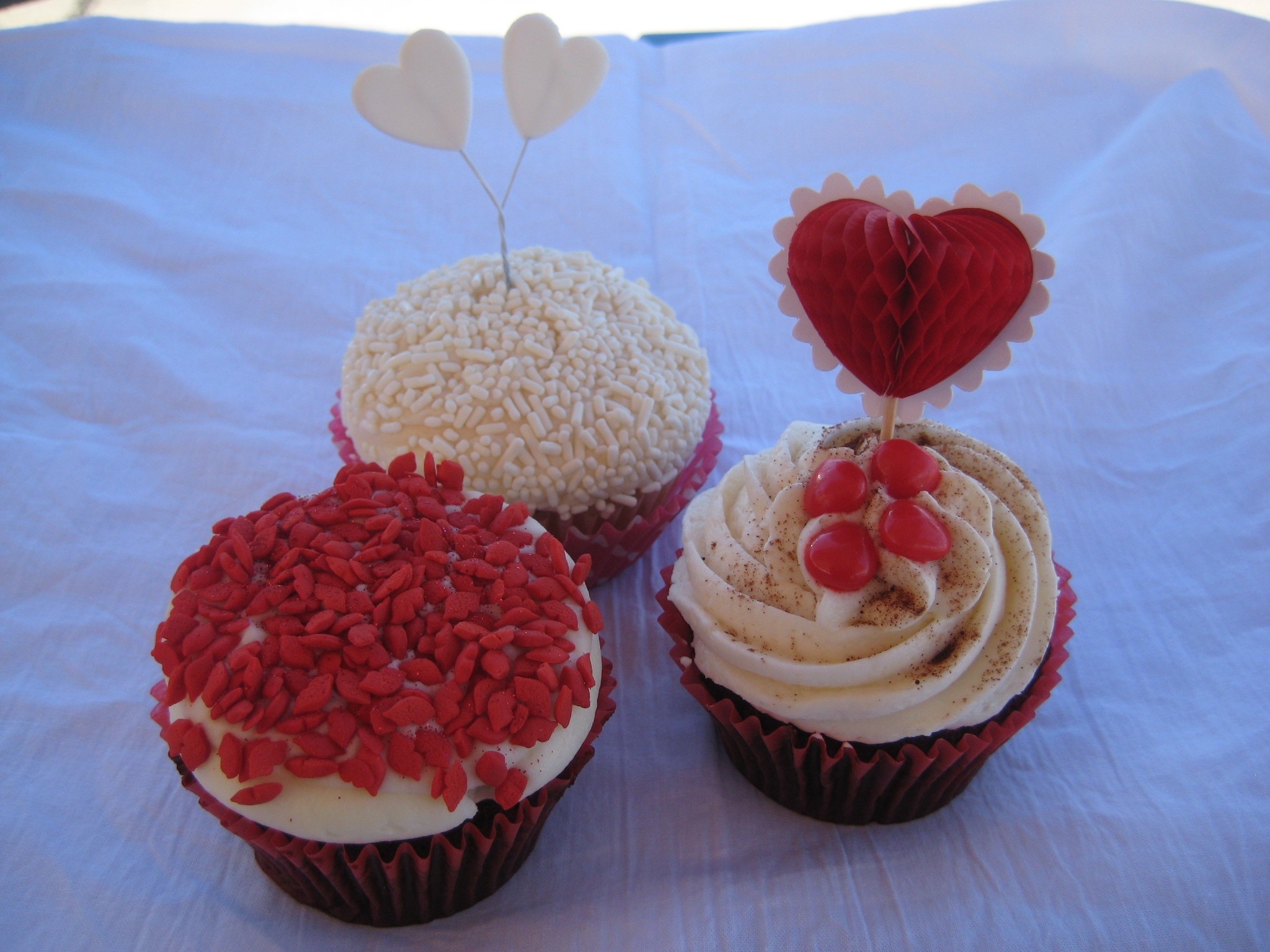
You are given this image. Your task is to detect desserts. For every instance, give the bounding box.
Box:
[150,452,616,927]
[654,417,1077,825]
[328,248,723,590]
